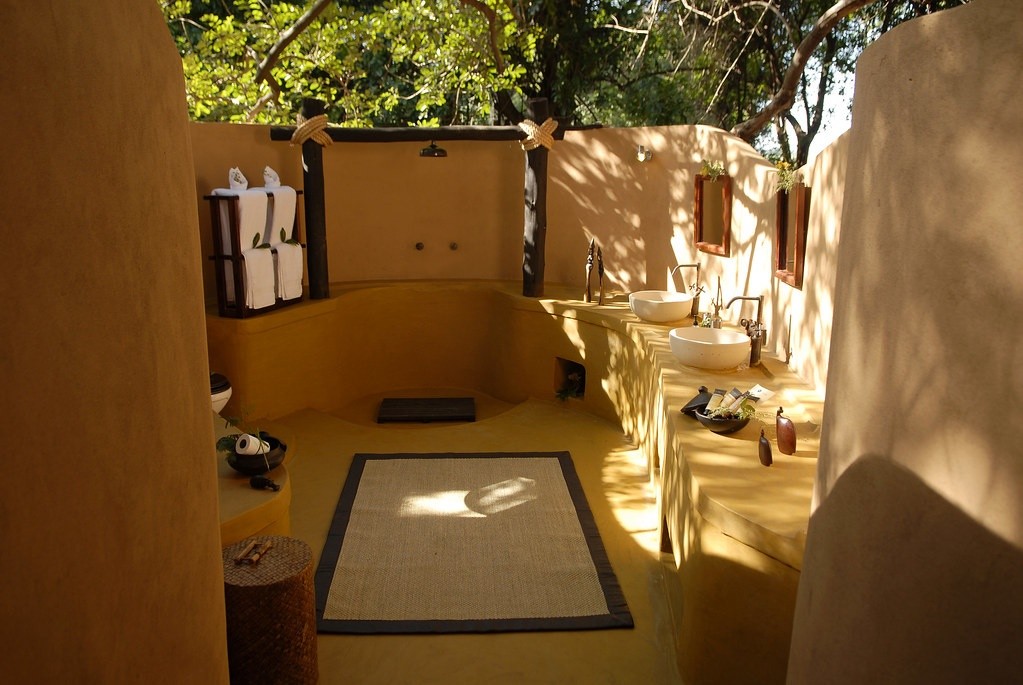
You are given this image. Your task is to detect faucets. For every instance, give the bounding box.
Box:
[726,295,764,366]
[670,263,699,316]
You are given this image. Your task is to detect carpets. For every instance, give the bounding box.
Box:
[314,452,635,634]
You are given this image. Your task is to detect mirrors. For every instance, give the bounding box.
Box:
[773,181,804,286]
[694,174,730,256]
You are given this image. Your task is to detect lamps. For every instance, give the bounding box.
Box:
[420,137,448,157]
[637,145,652,162]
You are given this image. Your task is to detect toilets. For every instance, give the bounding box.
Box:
[209,371,232,414]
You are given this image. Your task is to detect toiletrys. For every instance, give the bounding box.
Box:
[698,387,751,418]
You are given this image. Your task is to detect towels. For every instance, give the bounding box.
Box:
[210,166,276,310]
[255,167,304,302]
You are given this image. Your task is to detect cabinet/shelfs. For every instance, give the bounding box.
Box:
[204,191,308,319]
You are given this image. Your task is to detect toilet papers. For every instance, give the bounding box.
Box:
[235,433,271,454]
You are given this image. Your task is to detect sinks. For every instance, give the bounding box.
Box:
[626,290,691,321]
[668,327,751,370]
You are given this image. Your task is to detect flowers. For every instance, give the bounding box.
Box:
[776,161,793,194]
[700,159,728,181]
